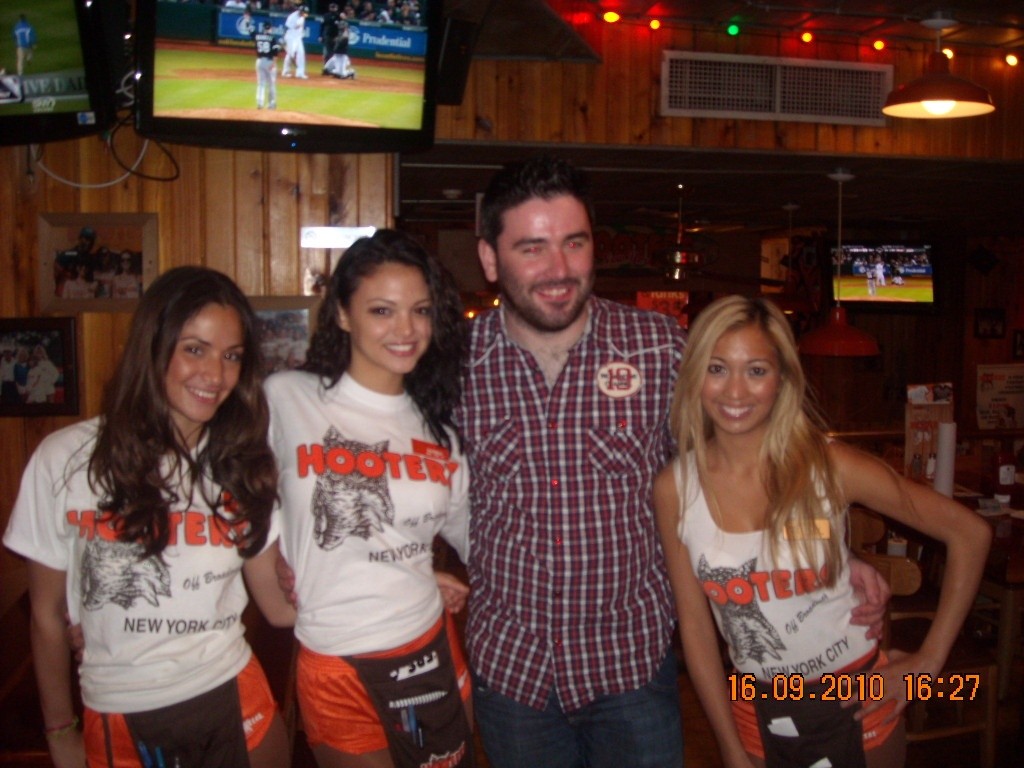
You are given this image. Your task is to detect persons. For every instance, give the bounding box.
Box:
[0,0,933,408]
[1,157,993,768]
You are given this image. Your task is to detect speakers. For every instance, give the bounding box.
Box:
[436,18,476,106]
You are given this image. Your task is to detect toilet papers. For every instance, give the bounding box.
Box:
[933,421,957,500]
[887,539,907,557]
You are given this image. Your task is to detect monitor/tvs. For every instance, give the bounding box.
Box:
[0,0,116,146]
[134,0,439,152]
[824,241,942,310]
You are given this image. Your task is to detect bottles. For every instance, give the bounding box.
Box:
[910,453,936,480]
[993,451,1017,504]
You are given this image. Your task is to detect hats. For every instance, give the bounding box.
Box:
[96,247,111,257]
[80,226,96,238]
[34,345,45,356]
[265,22,271,30]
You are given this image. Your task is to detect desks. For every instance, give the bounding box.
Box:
[921,463,1024,701]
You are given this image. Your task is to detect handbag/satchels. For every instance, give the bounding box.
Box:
[342,608,474,768]
[737,646,880,768]
[123,676,249,768]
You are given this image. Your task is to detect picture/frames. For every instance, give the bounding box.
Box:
[0,317,84,417]
[1012,331,1024,359]
[240,295,321,379]
[974,308,1006,338]
[33,212,157,311]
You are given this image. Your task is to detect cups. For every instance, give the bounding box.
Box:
[888,538,907,557]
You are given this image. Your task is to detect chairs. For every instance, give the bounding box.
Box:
[843,508,1002,768]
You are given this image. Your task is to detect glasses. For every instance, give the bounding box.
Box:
[119,257,131,262]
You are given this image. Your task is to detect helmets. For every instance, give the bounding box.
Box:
[299,4,309,11]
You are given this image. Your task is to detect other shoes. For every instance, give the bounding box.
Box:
[295,73,308,79]
[282,73,291,77]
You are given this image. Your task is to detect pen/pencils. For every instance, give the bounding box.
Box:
[401,704,423,748]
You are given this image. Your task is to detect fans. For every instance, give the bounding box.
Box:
[594,184,788,286]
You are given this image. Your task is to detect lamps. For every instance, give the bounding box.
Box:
[790,166,879,358]
[881,0,995,120]
